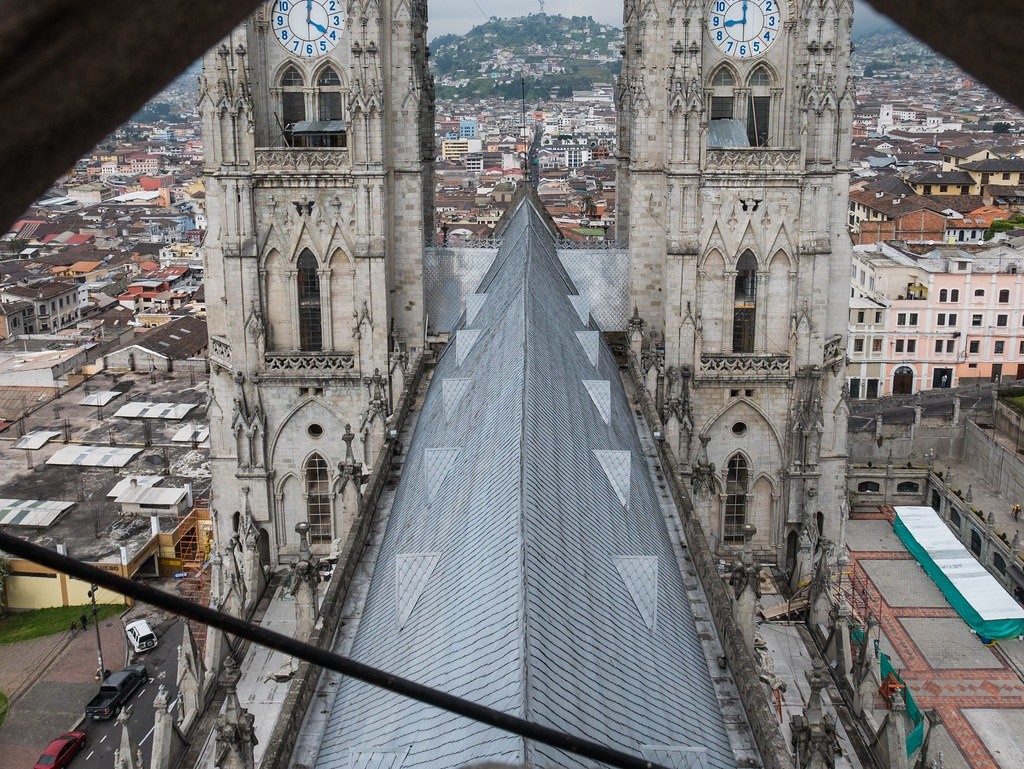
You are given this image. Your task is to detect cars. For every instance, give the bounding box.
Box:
[32,727,86,769]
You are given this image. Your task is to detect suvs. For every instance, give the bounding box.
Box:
[125,619,160,654]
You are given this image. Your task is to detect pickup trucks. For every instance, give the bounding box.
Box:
[84,662,148,722]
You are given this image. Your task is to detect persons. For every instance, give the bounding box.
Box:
[80,613,87,631]
[1012,504,1021,522]
[67,621,78,639]
